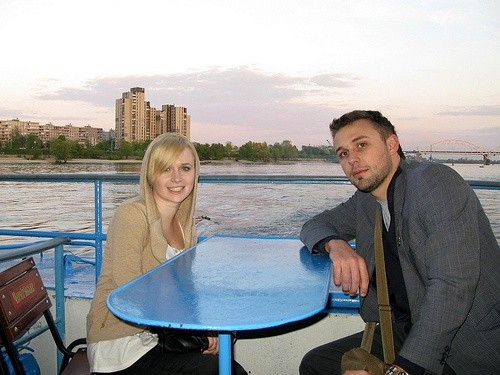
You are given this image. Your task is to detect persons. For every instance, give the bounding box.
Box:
[299,109,500,375]
[86,132,249,375]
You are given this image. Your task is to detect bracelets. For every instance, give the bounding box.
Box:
[385,364,410,375]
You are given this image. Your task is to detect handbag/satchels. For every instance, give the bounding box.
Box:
[341,347,388,375]
[147,326,208,353]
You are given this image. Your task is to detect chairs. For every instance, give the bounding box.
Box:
[0,256,91,375]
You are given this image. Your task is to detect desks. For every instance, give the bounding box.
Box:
[106,233,333,375]
